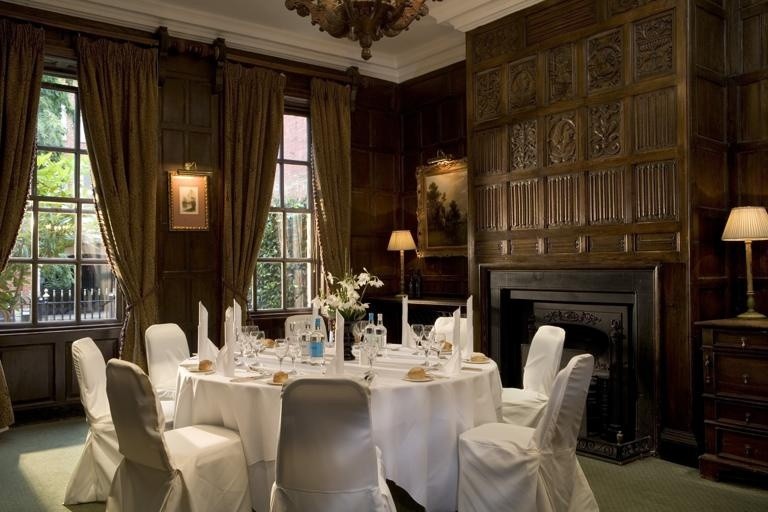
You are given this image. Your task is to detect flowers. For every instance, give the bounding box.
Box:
[309,266,385,322]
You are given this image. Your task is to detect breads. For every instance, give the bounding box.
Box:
[262,338,275,348]
[199,360,212,370]
[442,342,453,352]
[471,352,487,362]
[408,367,425,379]
[273,371,289,383]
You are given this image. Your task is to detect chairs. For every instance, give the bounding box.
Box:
[144,323,190,400]
[428,316,467,350]
[285,315,327,342]
[63,337,176,505]
[458,354,600,512]
[501,325,566,429]
[270,379,397,512]
[105,358,252,512]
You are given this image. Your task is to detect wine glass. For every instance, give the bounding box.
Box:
[363,341,377,375]
[320,356,331,379]
[234,323,299,375]
[351,319,368,367]
[409,322,451,370]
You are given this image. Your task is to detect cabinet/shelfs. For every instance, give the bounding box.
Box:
[693,319,768,491]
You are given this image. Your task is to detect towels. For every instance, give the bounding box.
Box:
[197,295,474,379]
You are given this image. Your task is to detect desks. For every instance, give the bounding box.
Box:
[368,296,467,307]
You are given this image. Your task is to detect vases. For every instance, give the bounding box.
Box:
[344,320,355,360]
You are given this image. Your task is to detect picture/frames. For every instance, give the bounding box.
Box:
[415,157,468,258]
[167,169,214,232]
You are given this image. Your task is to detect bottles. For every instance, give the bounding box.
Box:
[365,312,387,347]
[301,316,325,364]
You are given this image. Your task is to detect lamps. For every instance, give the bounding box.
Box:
[722,207,768,319]
[285,0,443,60]
[388,230,417,297]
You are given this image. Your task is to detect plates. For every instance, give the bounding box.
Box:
[267,378,292,387]
[467,359,490,365]
[440,347,459,355]
[189,368,212,373]
[404,376,433,382]
